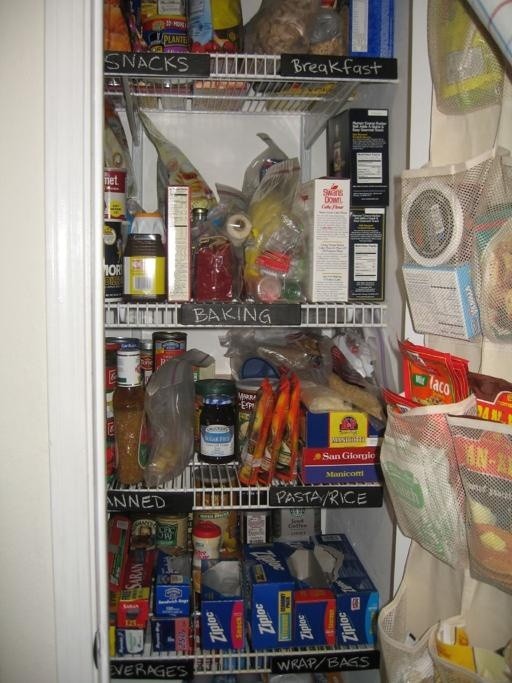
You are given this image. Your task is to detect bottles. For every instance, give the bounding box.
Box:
[114,340,147,487]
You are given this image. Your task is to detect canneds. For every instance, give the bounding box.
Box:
[105,331,187,482]
[155,514,187,550]
[193,464,239,554]
[121,232,166,302]
[102,167,126,299]
[198,393,236,466]
